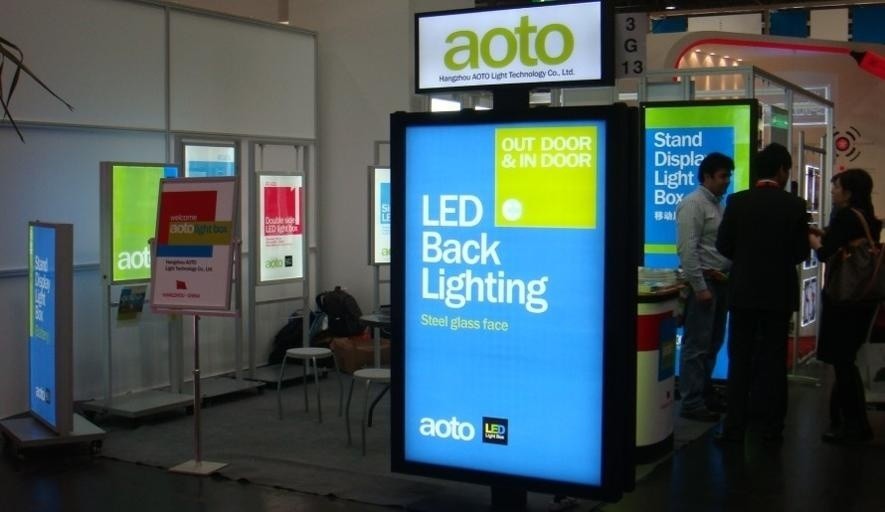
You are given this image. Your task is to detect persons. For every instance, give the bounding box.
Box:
[677,152,734,423]
[807,166,883,446]
[714,139,811,453]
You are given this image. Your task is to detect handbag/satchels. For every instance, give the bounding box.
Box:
[821,207,885,312]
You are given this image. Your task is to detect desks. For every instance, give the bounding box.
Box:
[360,314,391,327]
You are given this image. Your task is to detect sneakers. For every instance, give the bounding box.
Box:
[820,422,876,446]
[679,387,732,425]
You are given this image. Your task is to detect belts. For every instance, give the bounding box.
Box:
[703,265,729,284]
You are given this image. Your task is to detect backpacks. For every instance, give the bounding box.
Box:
[315,284,366,340]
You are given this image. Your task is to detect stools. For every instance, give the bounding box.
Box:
[345,368,392,456]
[276,348,345,423]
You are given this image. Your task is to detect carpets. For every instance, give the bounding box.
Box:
[92,370,729,512]
